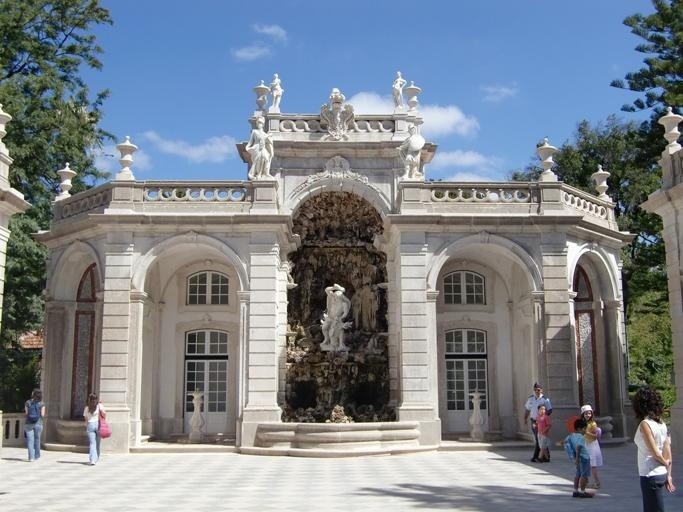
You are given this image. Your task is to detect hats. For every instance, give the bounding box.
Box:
[581,404,593,414]
[534,382,542,388]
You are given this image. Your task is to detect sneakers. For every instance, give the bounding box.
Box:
[573,481,600,497]
[531,457,550,463]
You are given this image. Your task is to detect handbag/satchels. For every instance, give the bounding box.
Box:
[99,411,111,437]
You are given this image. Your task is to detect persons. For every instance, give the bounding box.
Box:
[628,387,675,512]
[522,381,552,461]
[82,392,112,465]
[317,283,353,350]
[391,70,407,106]
[579,404,603,489]
[561,418,595,498]
[246,115,273,178]
[532,403,552,463]
[267,73,284,107]
[23,389,44,462]
[395,123,425,178]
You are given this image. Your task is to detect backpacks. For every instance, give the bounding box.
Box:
[28,403,40,423]
[564,441,577,464]
